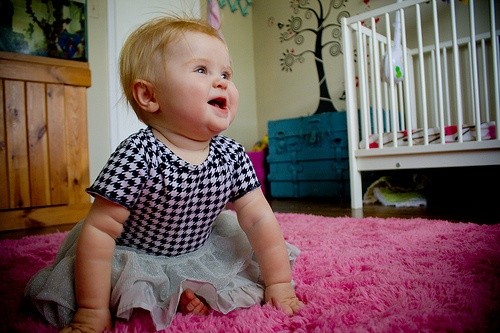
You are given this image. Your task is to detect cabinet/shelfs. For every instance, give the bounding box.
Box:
[0,51,92,240]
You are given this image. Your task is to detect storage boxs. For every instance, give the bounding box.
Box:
[225,151,269,208]
[267,107,402,202]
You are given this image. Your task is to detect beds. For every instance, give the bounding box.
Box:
[340,0,500,210]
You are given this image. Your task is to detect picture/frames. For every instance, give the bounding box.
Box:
[0,0,89,64]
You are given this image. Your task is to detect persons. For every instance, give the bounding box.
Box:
[21,16,304,333]
